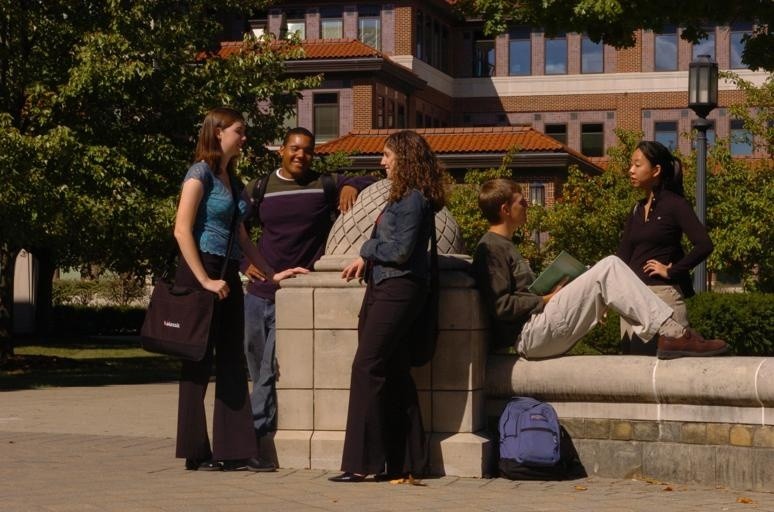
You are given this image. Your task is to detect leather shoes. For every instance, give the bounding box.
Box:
[655,329,728,360]
[184,445,277,471]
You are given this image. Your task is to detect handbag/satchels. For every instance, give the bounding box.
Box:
[401,285,438,367]
[139,277,215,363]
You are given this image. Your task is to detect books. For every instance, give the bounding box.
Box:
[527,250,588,296]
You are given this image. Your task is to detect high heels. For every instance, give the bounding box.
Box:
[328,459,424,483]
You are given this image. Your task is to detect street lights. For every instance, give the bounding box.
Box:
[686,52,720,294]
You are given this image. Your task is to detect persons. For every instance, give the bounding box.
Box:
[467,176,731,362]
[609,139,714,354]
[230,130,377,450]
[170,108,279,472]
[328,130,446,483]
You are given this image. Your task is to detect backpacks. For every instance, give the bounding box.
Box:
[498,394,568,480]
